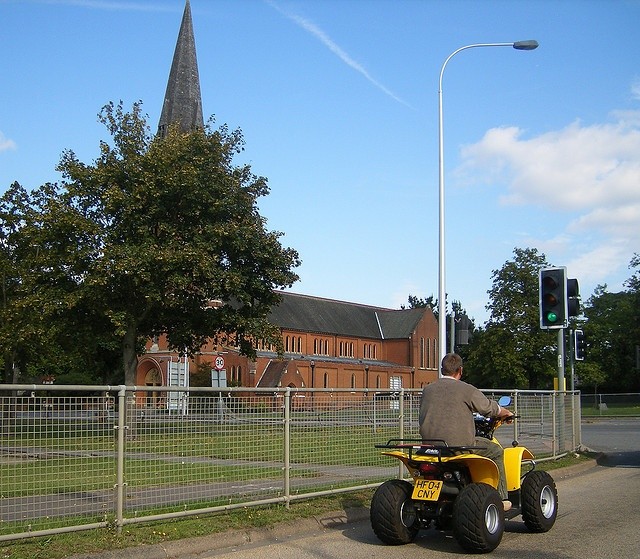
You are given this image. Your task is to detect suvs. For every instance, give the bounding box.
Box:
[370,395,558,553]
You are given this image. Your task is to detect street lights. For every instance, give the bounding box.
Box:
[437,39,539,377]
[310,359,315,411]
[365,364,369,395]
[411,368,415,399]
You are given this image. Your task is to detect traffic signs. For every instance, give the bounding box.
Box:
[214,356,225,369]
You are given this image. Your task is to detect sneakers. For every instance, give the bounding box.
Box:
[502,500,512,512]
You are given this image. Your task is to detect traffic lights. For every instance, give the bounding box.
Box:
[570,278,584,318]
[575,330,585,360]
[540,267,568,329]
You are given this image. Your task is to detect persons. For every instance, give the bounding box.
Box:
[418,353,514,512]
